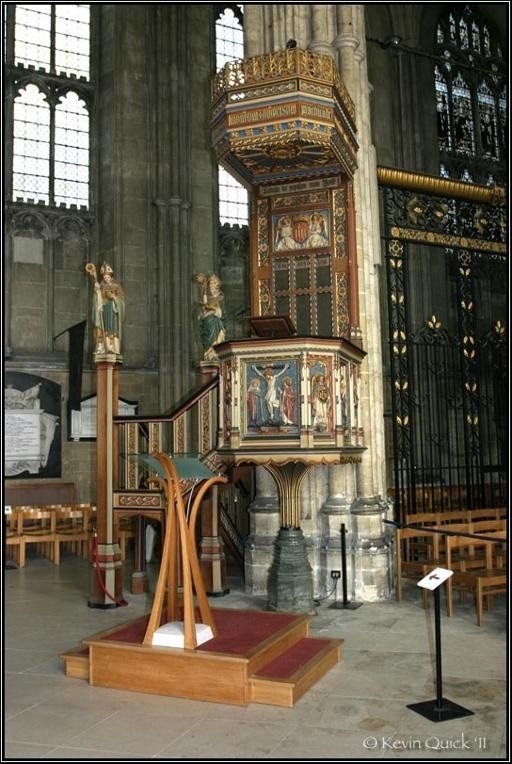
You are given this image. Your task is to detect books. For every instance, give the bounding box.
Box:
[417,566,454,592]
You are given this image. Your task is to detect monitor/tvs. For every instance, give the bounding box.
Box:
[249,314,296,337]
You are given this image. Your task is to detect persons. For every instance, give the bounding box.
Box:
[310,372,332,431]
[247,378,264,425]
[275,215,303,251]
[86,259,127,355]
[277,377,296,426]
[192,271,226,363]
[250,362,291,421]
[303,214,330,249]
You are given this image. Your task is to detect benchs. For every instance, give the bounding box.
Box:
[396,506,508,626]
[5,501,96,568]
